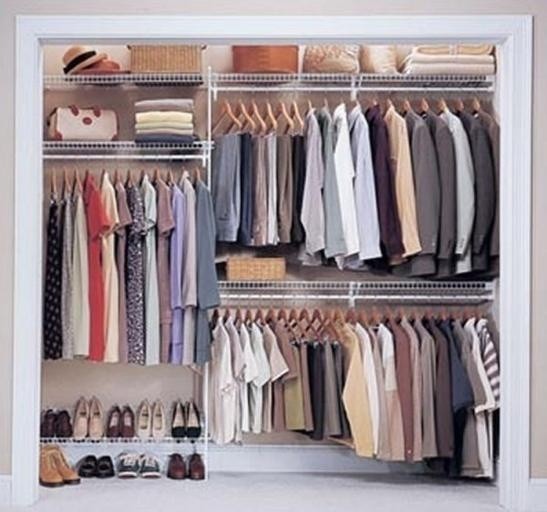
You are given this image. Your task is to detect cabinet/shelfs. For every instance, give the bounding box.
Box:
[39,40,502,495]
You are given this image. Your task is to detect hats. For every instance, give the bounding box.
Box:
[60,44,131,87]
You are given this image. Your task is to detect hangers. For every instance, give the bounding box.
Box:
[47,152,201,198]
[213,291,493,343]
[213,86,485,136]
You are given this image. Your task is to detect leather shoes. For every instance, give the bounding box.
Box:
[72,395,107,444]
[105,404,136,445]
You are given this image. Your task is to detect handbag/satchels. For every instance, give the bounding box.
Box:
[46,103,120,142]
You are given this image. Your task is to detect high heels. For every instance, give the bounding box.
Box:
[37,445,81,489]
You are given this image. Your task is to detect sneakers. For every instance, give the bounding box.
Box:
[166,452,205,482]
[76,453,116,480]
[40,406,74,445]
[116,450,164,481]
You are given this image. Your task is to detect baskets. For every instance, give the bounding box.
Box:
[126,43,208,88]
[223,257,288,286]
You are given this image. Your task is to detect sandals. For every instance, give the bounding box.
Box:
[170,398,202,445]
[133,398,167,445]
